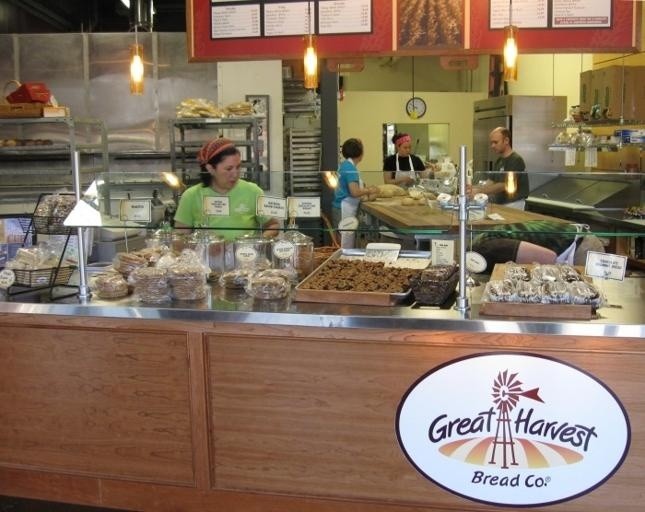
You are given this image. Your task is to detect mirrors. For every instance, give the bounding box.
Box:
[382,122,449,167]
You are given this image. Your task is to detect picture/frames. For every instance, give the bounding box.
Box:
[246,93,270,192]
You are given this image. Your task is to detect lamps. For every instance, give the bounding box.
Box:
[129,1,145,97]
[303,1,319,90]
[503,0,520,81]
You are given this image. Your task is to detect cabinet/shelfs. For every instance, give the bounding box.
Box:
[169,118,267,206]
[545,119,644,154]
[579,65,645,123]
[1,116,200,216]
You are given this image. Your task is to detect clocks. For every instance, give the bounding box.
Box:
[405,97,427,120]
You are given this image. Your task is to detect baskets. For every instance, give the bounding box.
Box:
[0,265,77,288]
[18,215,88,235]
[18,83,45,90]
[0,81,42,118]
[7,90,49,103]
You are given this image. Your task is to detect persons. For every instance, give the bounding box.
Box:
[465,126,529,211]
[465,220,606,274]
[331,138,377,249]
[381,132,425,185]
[173,138,280,239]
